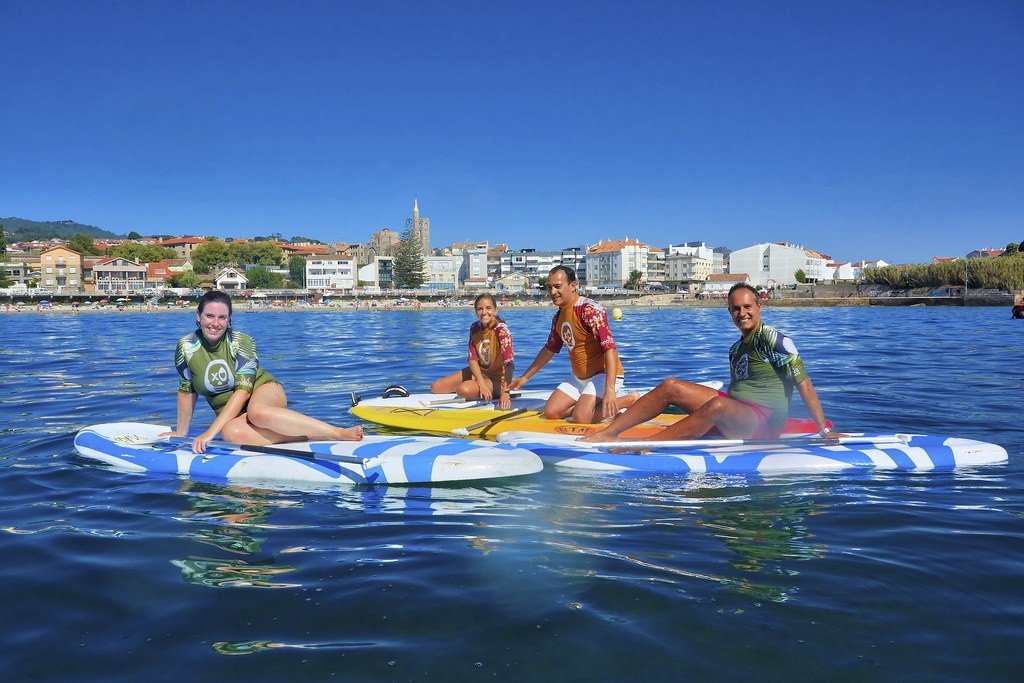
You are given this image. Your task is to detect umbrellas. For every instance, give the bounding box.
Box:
[288,299,306,303]
[175,299,191,304]
[166,301,174,305]
[100,299,108,302]
[324,298,334,302]
[649,284,670,290]
[84,301,91,305]
[39,299,49,307]
[17,301,26,305]
[272,299,284,303]
[116,297,143,306]
[72,301,80,305]
[153,302,160,304]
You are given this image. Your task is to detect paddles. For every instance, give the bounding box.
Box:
[509,431,915,454]
[446,400,552,441]
[416,387,553,412]
[100,422,392,474]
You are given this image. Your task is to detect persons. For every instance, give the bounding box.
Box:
[574,282,851,456]
[1011,296,1024,318]
[71,308,79,316]
[430,292,516,411]
[158,291,365,454]
[503,264,641,424]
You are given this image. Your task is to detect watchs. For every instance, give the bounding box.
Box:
[817,426,833,438]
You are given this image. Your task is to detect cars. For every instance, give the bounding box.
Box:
[650,284,670,291]
[676,288,690,294]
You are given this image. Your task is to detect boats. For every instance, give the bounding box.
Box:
[1011,301,1024,319]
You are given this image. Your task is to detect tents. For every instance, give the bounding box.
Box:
[699,291,729,299]
[758,288,768,294]
